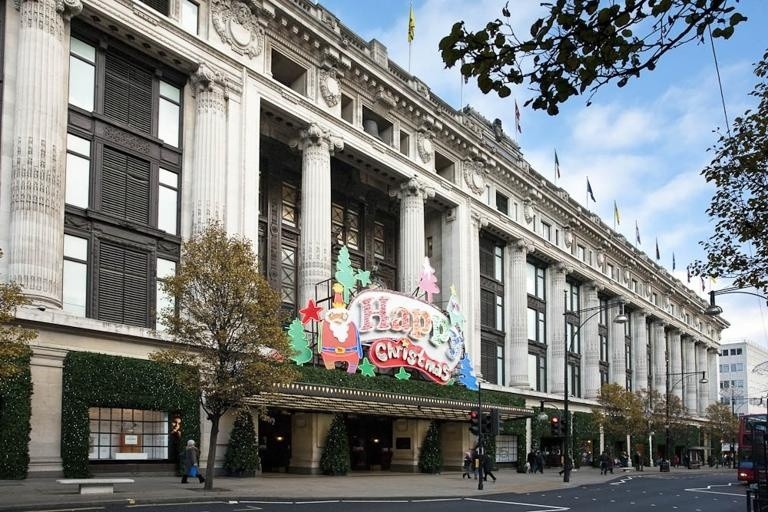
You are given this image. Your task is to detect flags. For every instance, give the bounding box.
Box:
[655,238,659,261]
[700,276,704,293]
[686,266,690,283]
[614,200,620,227]
[513,98,522,136]
[635,219,641,246]
[407,0,416,45]
[586,175,595,204]
[459,45,469,85]
[672,250,675,271]
[553,147,560,179]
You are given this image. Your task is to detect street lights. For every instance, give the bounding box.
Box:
[478,379,487,490]
[660,359,708,472]
[563,299,631,483]
[731,398,762,470]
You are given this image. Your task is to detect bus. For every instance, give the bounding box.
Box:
[737,414,768,486]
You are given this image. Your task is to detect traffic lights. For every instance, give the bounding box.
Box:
[469,407,491,435]
[551,415,567,438]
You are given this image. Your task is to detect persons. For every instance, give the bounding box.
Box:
[168,416,181,438]
[481,451,497,482]
[461,455,472,480]
[524,447,737,476]
[471,450,479,480]
[180,439,206,486]
